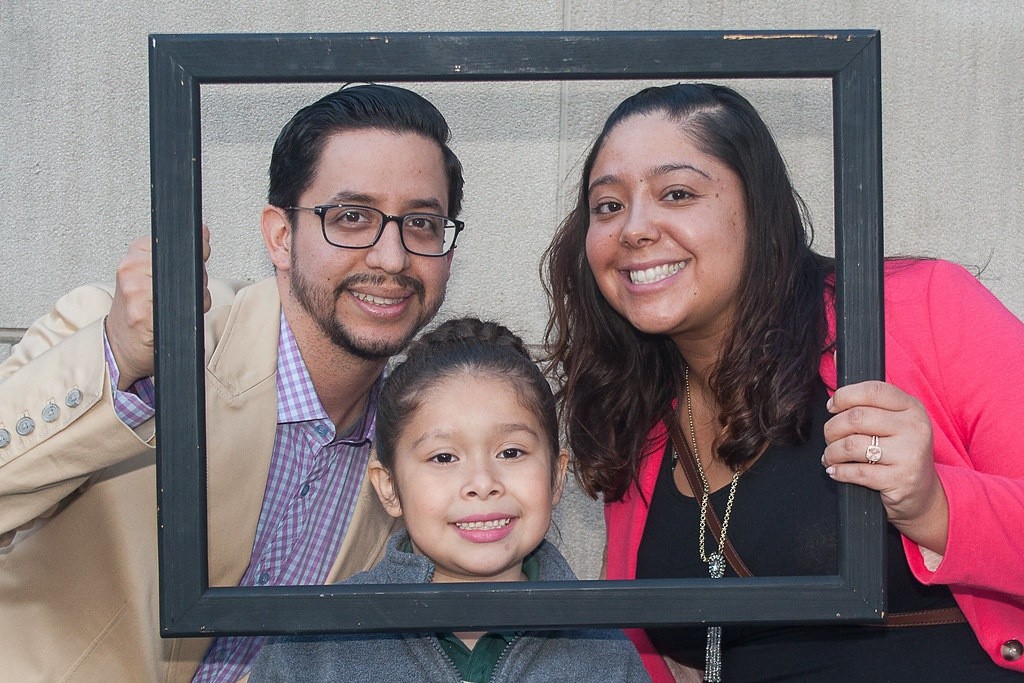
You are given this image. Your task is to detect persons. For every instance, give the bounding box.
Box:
[0,88,466,683]
[250,318,650,683]
[545,86,1024,683]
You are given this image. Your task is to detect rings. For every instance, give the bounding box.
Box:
[866,433,882,464]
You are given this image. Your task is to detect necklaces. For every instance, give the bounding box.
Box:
[685,364,739,580]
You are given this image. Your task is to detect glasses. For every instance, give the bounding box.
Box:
[283,204,465,257]
